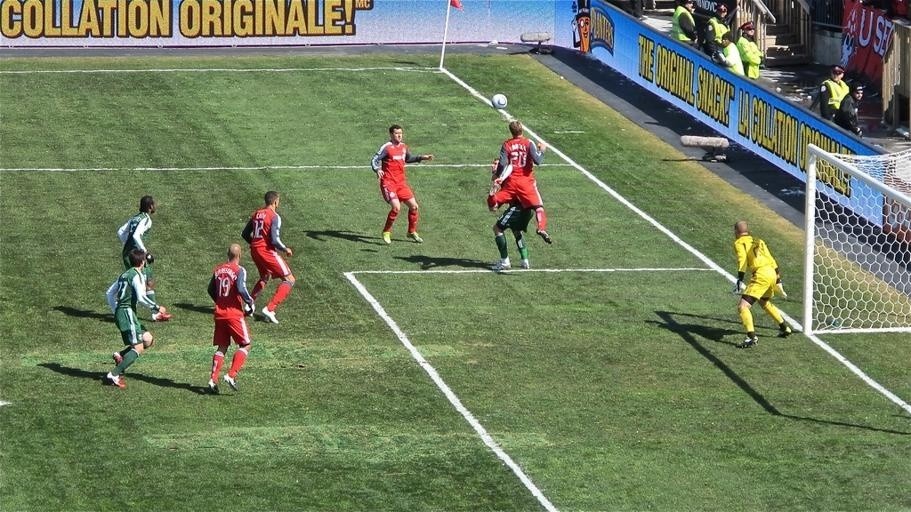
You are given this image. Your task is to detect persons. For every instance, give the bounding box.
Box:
[819,64,851,122]
[371,125,433,244]
[240,190,295,325]
[490,157,530,271]
[206,243,256,395]
[671,0,764,80]
[105,248,166,388]
[732,220,792,348]
[116,194,172,322]
[835,81,863,139]
[487,120,553,245]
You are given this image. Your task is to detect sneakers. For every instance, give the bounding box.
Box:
[262,306,279,323]
[778,327,791,336]
[407,232,423,242]
[106,353,125,387]
[536,229,552,244]
[151,313,171,321]
[492,259,530,269]
[382,231,391,244]
[208,374,238,393]
[738,337,758,347]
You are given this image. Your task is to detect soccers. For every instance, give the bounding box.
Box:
[493,94,508,108]
